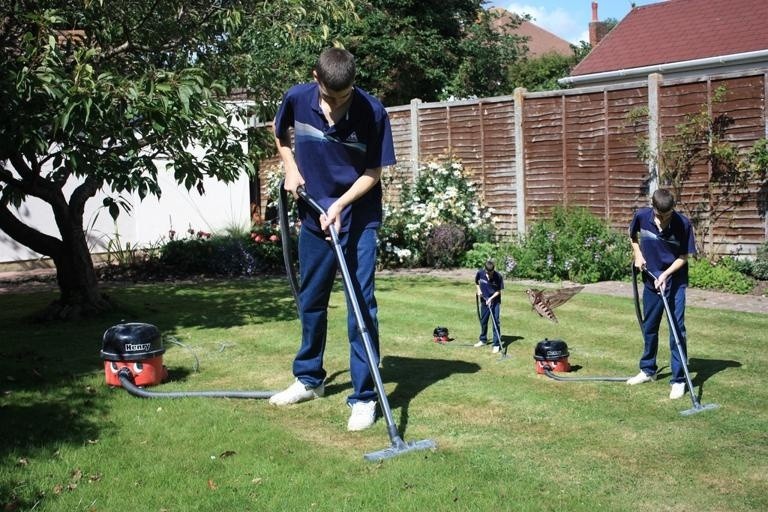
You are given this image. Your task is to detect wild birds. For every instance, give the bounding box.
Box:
[525,286,585,324]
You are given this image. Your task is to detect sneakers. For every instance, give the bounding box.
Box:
[627,369,657,386]
[474,340,488,348]
[268,378,325,406]
[347,400,377,431]
[491,345,500,354]
[670,382,685,399]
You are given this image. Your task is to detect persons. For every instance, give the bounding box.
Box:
[269,49,397,431]
[474,262,504,353]
[626,188,696,399]
